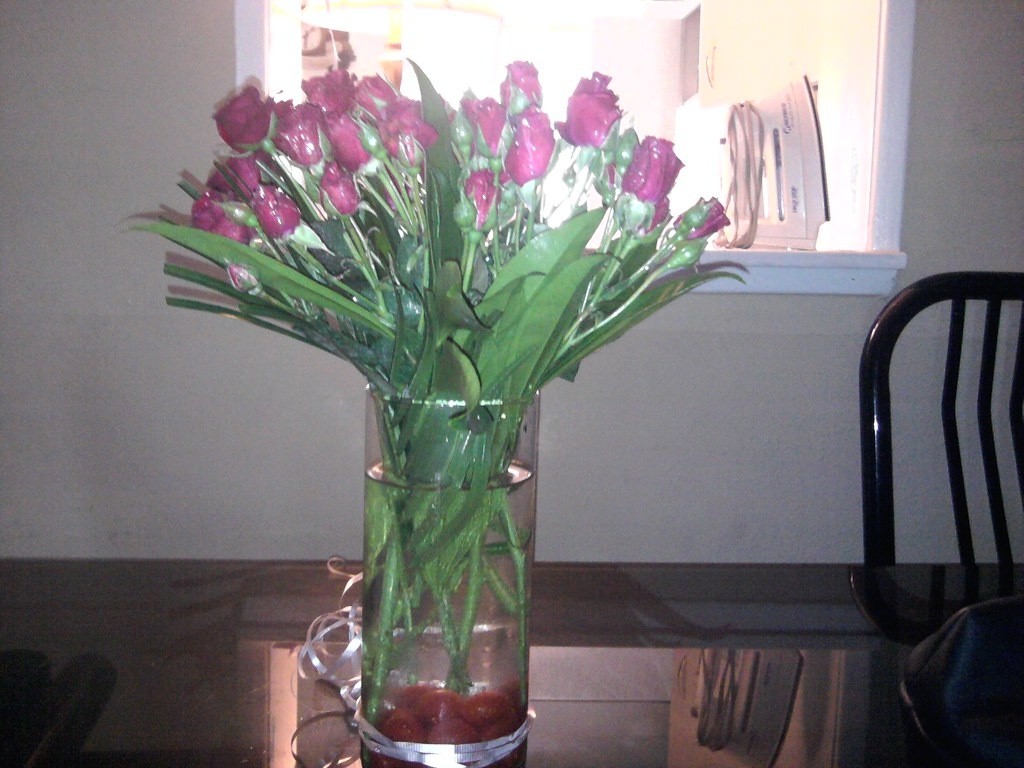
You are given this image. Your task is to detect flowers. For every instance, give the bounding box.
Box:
[121,32,749,757]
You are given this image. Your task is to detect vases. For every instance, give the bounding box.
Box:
[358,380,542,766]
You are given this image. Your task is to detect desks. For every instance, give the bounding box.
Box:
[2,550,1022,767]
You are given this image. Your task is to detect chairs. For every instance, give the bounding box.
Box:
[857,265,1024,561]
